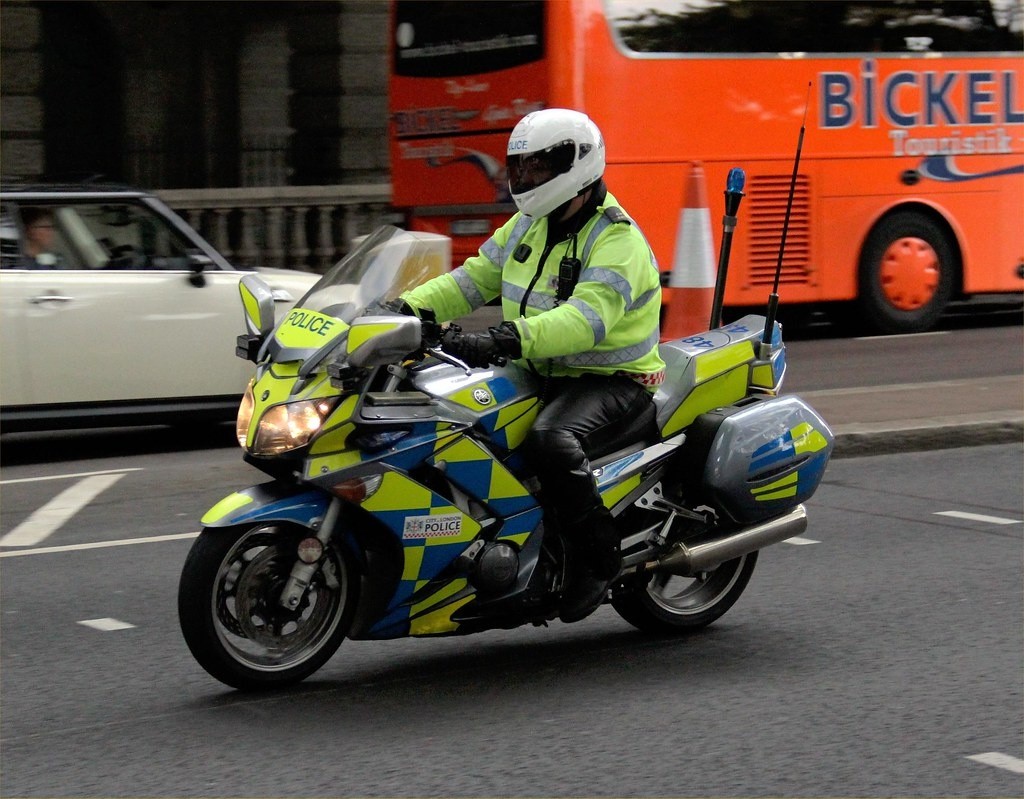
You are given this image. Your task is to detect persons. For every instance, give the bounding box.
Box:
[18,207,67,270]
[382,108,662,623]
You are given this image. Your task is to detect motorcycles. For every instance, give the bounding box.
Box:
[175,78,836,693]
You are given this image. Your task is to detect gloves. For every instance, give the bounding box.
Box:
[441,321,523,370]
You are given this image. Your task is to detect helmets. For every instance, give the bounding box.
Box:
[506,106,607,217]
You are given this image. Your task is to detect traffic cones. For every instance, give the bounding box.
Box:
[661,159,723,342]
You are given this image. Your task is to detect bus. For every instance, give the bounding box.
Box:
[385,0,1024,338]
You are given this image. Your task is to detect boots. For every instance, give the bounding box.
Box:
[549,462,622,622]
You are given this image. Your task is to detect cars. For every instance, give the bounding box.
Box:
[0,190,365,435]
